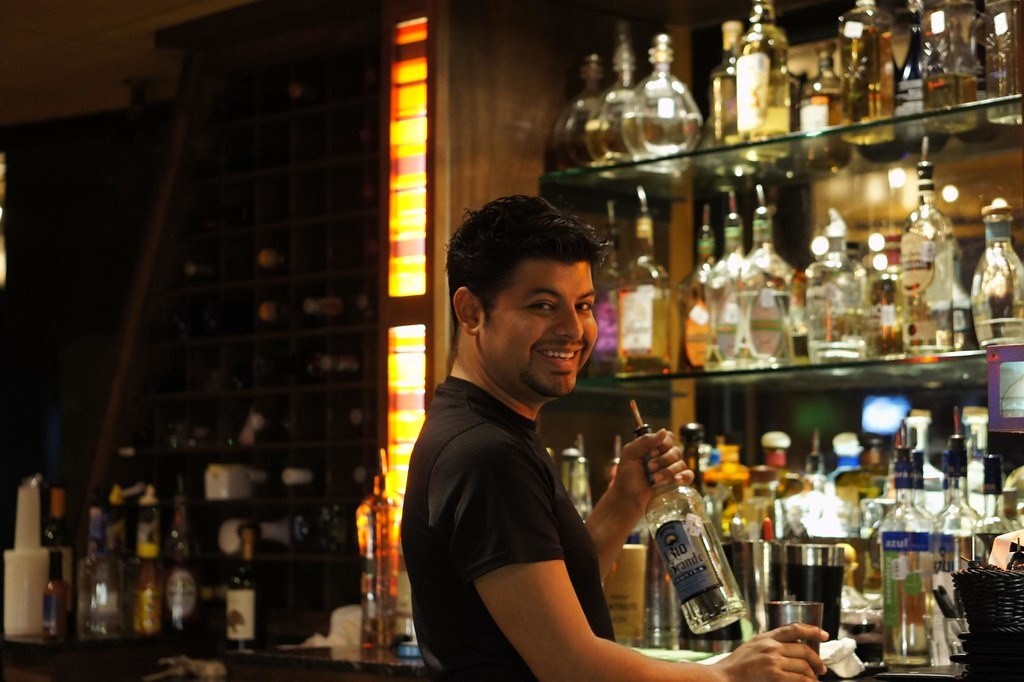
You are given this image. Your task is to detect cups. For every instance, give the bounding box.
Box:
[680,538,845,660]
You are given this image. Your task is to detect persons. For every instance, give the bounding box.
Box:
[395,192,832,682]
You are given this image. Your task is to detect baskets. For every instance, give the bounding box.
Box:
[950,565,1024,636]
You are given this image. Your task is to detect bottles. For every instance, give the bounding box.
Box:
[226,528,257,649]
[41,464,76,638]
[77,484,195,641]
[42,552,67,637]
[544,0,1024,171]
[583,162,1024,384]
[544,419,1023,667]
[355,471,422,660]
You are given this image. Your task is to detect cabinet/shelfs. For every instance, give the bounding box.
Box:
[70,0,387,604]
[538,92,1024,395]
